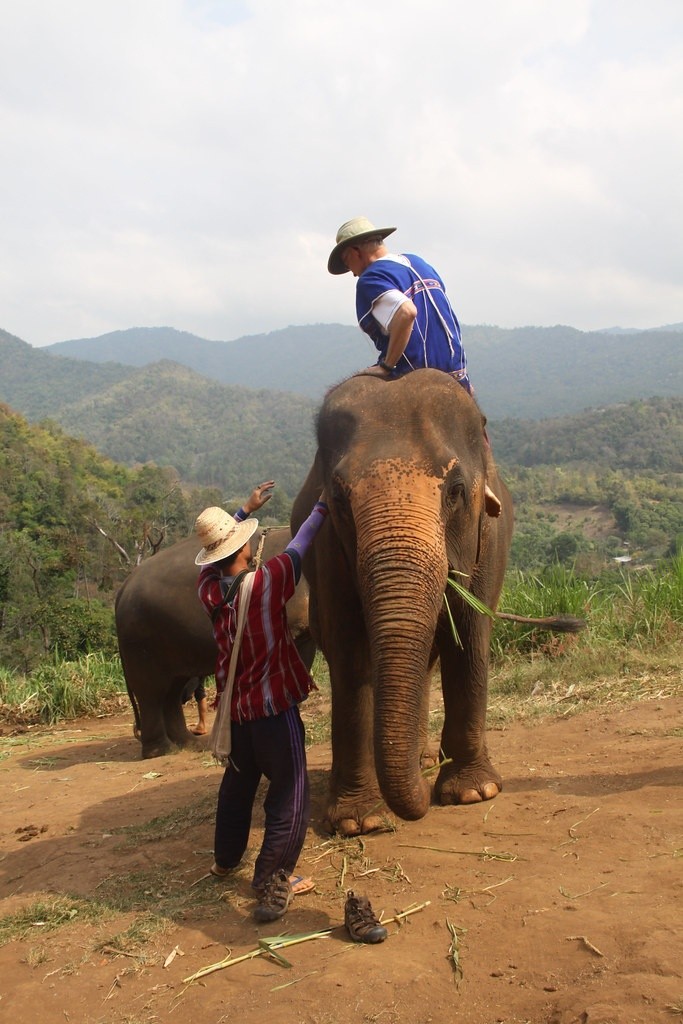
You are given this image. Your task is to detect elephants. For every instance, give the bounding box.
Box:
[289,368,586,838]
[115,526,315,760]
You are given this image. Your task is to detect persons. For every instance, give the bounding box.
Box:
[327,218,502,518]
[193,480,329,894]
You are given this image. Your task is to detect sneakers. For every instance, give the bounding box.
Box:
[343,890,388,944]
[255,869,292,921]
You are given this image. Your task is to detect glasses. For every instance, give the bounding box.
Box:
[341,249,351,272]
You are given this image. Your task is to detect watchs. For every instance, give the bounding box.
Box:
[378,357,397,372]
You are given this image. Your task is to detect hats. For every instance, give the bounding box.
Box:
[194,506,259,566]
[328,216,397,275]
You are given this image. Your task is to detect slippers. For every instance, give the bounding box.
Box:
[288,875,317,895]
[208,862,237,877]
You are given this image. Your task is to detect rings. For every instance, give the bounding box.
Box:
[258,486,261,489]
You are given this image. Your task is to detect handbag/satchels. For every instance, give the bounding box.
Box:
[208,692,232,768]
